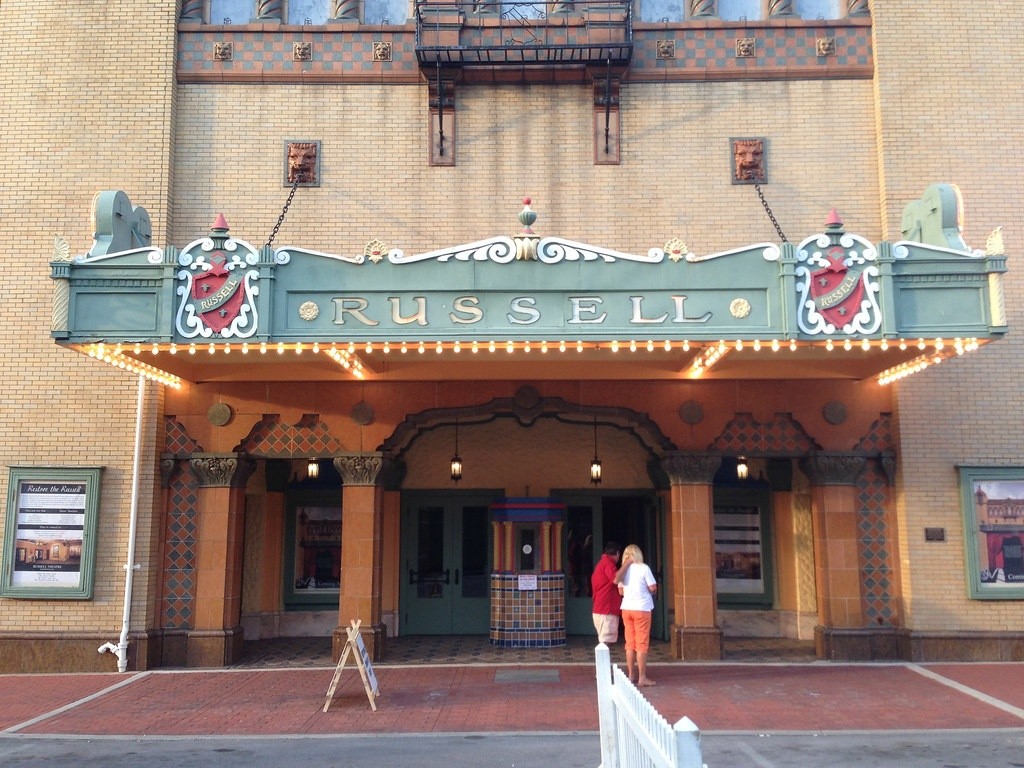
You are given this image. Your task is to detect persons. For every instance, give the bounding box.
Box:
[591,541,633,680]
[617,543,657,686]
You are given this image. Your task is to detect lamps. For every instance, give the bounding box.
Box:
[589,413,601,488]
[307,457,319,477]
[450,413,462,484]
[735,457,749,480]
